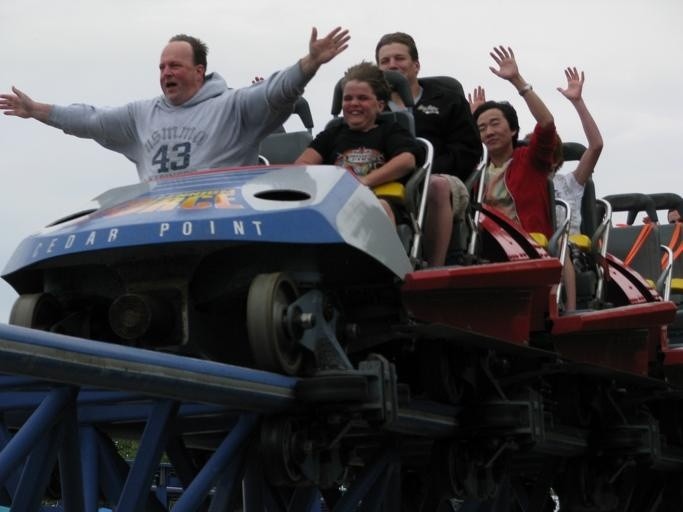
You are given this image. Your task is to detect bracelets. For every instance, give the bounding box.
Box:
[519,83,533,96]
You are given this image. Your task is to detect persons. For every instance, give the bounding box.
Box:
[0,24,353,184]
[468,64,605,313]
[642,209,681,225]
[374,30,482,271]
[468,43,559,263]
[292,61,424,233]
[247,76,286,133]
